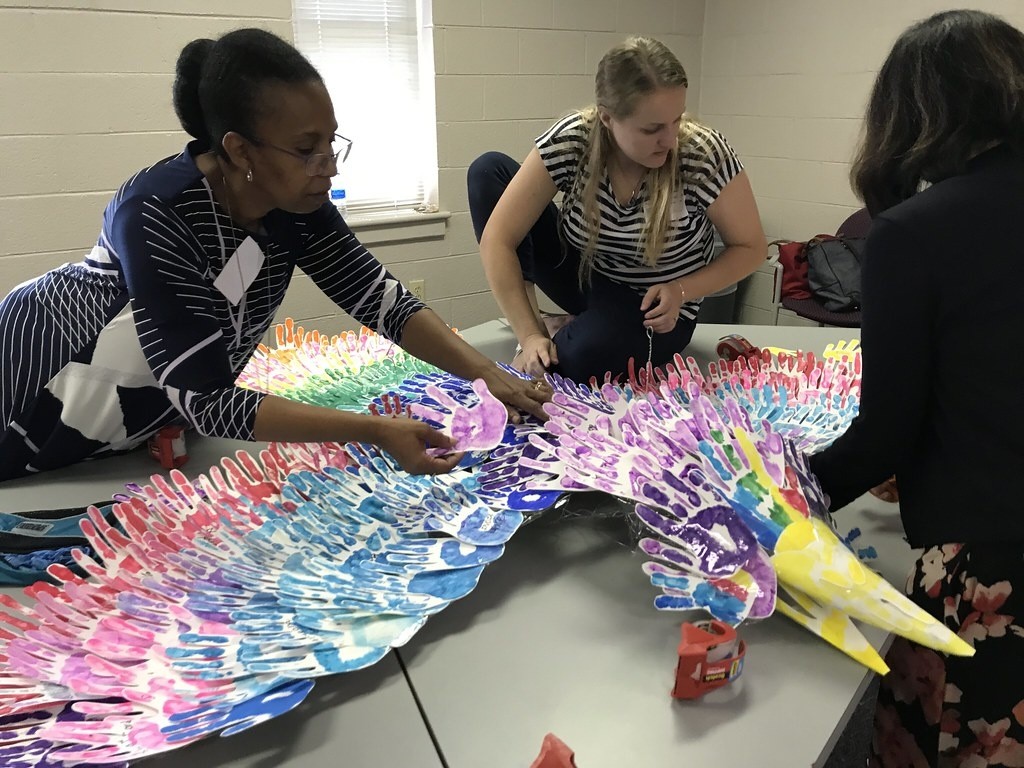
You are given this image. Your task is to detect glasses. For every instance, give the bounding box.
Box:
[241,133,352,176]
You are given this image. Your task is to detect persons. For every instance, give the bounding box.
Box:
[468,35,770,389]
[0,30,554,485]
[810,9,1024,768]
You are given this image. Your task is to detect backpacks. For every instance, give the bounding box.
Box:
[807,235,866,312]
[767,240,814,299]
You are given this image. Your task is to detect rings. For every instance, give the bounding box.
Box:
[673,316,678,322]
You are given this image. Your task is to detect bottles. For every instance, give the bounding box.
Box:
[330,173,346,218]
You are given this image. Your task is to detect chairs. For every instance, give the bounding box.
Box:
[770,205,877,328]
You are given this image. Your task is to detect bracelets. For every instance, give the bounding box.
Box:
[675,277,686,305]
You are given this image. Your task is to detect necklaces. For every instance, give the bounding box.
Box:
[218,173,271,394]
[611,153,646,205]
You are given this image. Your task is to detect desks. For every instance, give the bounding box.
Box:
[0,314,922,768]
